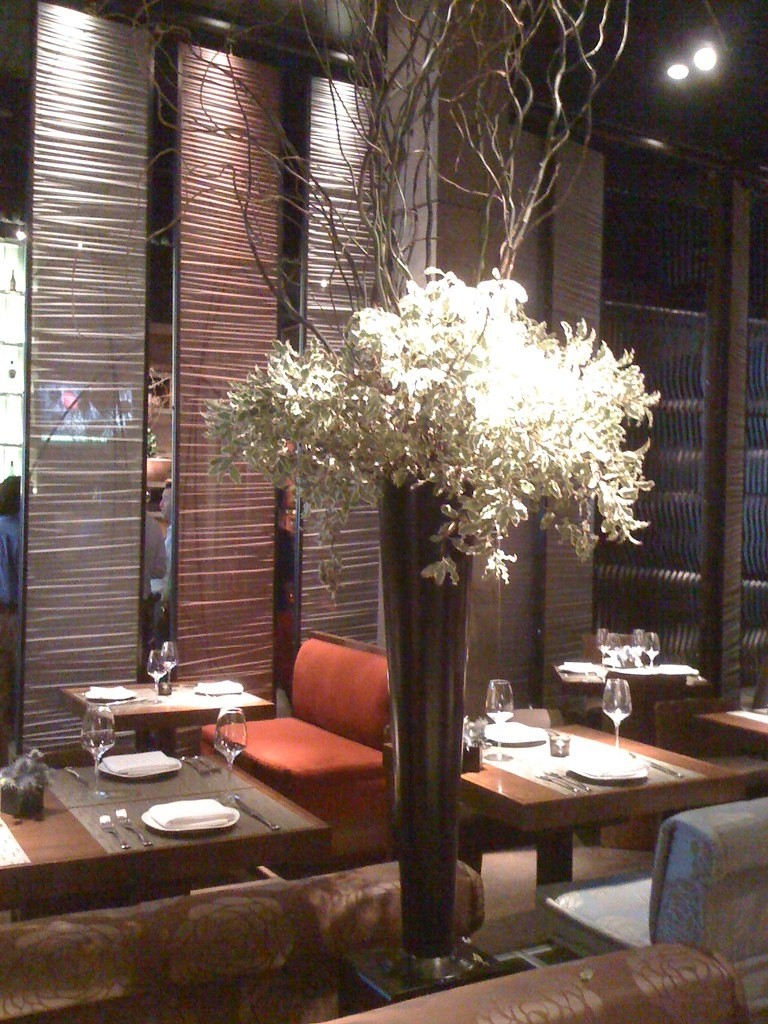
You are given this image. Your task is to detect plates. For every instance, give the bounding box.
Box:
[567,754,649,782]
[141,804,242,832]
[98,754,182,778]
[85,690,137,699]
[195,680,244,695]
[485,723,547,744]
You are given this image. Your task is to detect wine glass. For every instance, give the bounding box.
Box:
[79,703,117,799]
[484,680,514,762]
[145,642,179,704]
[595,626,661,675]
[601,679,633,748]
[214,708,246,804]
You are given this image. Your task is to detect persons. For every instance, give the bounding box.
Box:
[142,478,173,657]
[0,475,20,745]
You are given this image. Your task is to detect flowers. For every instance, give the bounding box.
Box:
[202,265,659,580]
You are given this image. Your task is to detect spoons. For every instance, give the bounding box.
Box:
[99,815,131,849]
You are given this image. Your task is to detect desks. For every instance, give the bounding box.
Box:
[459,723,747,890]
[551,663,712,747]
[695,711,768,746]
[0,756,333,910]
[58,681,276,756]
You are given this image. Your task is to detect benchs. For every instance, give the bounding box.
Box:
[0,858,484,1015]
[199,631,392,879]
[534,800,768,1013]
[330,942,749,1024]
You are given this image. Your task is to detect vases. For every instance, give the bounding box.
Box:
[375,474,476,963]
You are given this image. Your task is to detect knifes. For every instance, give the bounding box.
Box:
[64,766,98,792]
[232,795,282,831]
[639,753,684,777]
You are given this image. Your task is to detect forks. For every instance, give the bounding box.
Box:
[535,767,591,793]
[114,809,152,846]
[181,753,222,775]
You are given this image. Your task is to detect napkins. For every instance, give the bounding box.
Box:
[151,799,236,830]
[86,685,136,702]
[103,750,181,776]
[195,678,243,695]
